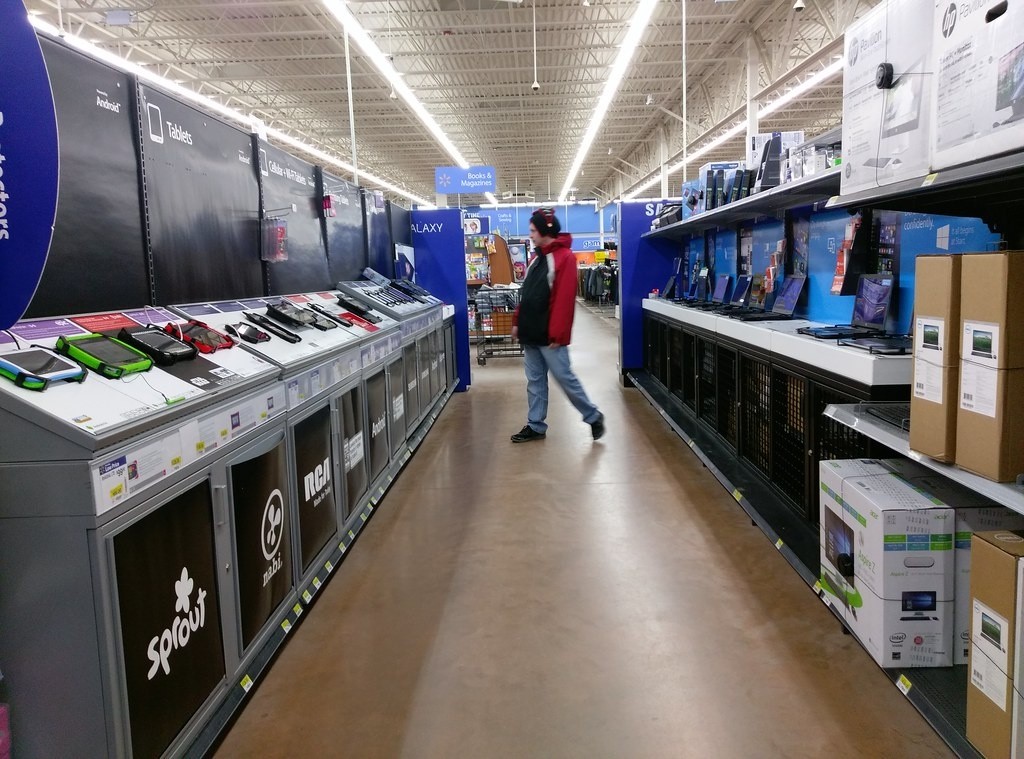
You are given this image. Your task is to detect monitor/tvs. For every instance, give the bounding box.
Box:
[973,330,992,353]
[825,504,854,589]
[880,54,926,154]
[923,325,939,345]
[995,42,1024,125]
[982,612,1001,644]
[902,591,936,616]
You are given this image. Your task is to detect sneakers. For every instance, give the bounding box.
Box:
[591,412,605,440]
[511,425,546,442]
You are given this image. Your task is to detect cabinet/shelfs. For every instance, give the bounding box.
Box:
[466,277,491,340]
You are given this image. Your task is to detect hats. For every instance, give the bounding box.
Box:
[530,214,561,237]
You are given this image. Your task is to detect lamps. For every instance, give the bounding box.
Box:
[793,0,805,13]
[530,0,541,92]
[608,147,614,154]
[645,94,655,105]
[57,0,65,39]
[389,85,398,100]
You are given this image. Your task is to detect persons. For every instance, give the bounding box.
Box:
[510,208,605,443]
[470,222,477,232]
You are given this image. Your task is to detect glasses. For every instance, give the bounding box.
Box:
[532,207,554,227]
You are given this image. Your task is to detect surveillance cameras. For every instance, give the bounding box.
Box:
[793,1,805,13]
[532,82,540,92]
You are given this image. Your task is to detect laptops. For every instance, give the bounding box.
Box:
[661,267,917,353]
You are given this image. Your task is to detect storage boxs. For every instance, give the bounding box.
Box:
[665,128,1002,354]
[908,252,963,464]
[928,0,1024,173]
[880,455,1024,666]
[954,250,1024,484]
[839,0,931,196]
[815,455,958,677]
[961,525,1024,759]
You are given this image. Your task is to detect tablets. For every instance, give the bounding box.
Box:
[0,319,233,383]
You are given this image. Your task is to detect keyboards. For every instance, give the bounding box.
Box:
[980,633,1001,651]
[900,617,930,621]
[863,158,891,168]
[971,351,992,358]
[923,344,939,350]
[824,571,849,609]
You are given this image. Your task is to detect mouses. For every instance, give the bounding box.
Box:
[940,347,942,350]
[993,355,996,359]
[932,616,938,620]
[852,606,856,616]
[1002,648,1005,653]
[893,159,903,169]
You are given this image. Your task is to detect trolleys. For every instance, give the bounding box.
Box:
[470,281,531,365]
